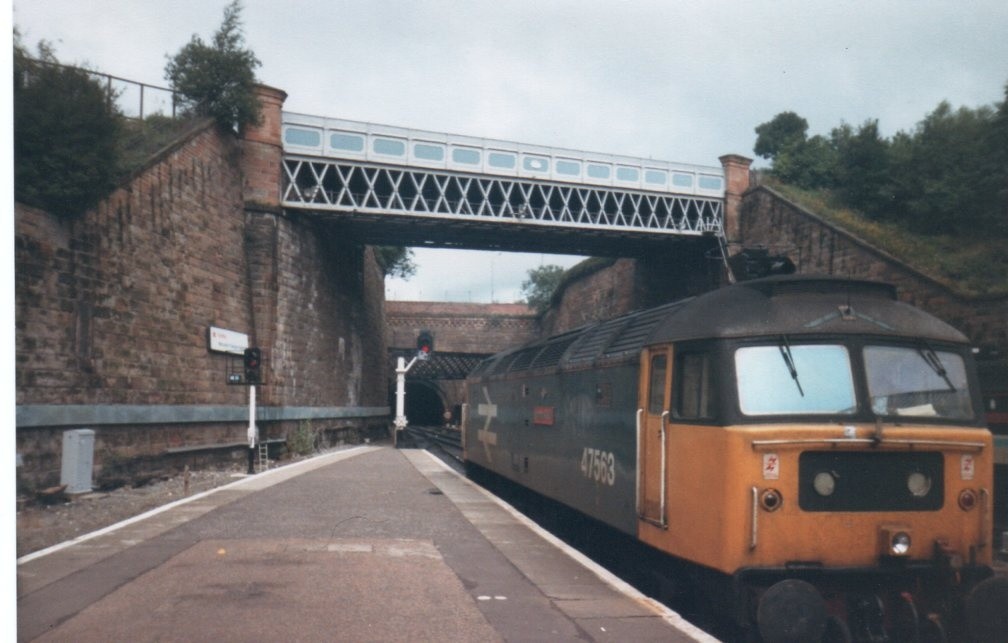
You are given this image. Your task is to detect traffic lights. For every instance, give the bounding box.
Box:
[243,347,262,383]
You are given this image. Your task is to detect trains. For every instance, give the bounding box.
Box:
[460,244,996,642]
